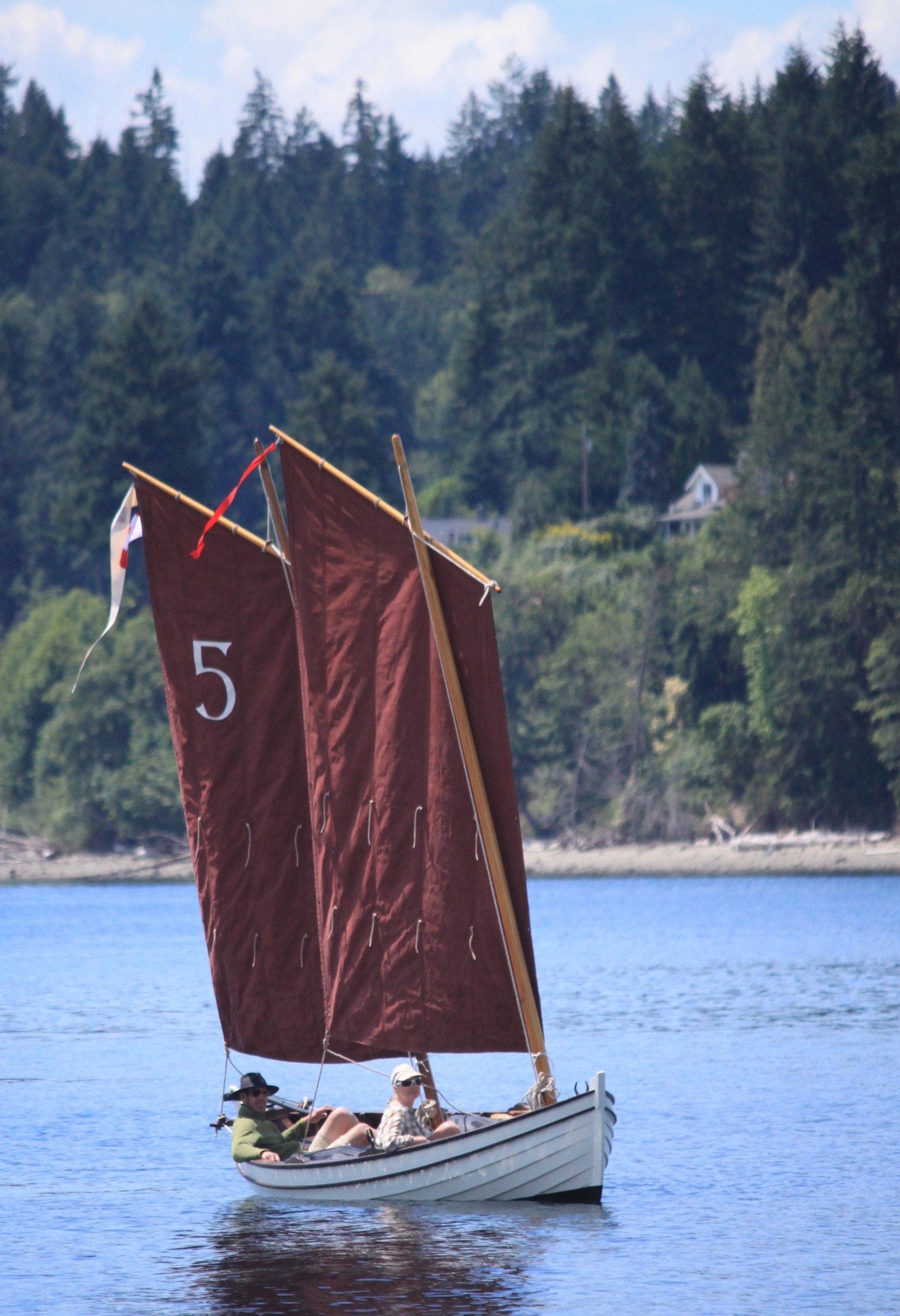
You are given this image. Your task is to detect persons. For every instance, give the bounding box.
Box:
[374,1064,461,1152]
[231,1072,379,1164]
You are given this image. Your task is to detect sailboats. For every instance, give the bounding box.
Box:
[67,420,617,1208]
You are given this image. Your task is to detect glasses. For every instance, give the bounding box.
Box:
[252,1090,269,1097]
[395,1077,424,1087]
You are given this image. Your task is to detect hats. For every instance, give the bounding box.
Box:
[391,1064,427,1085]
[223,1072,279,1101]
